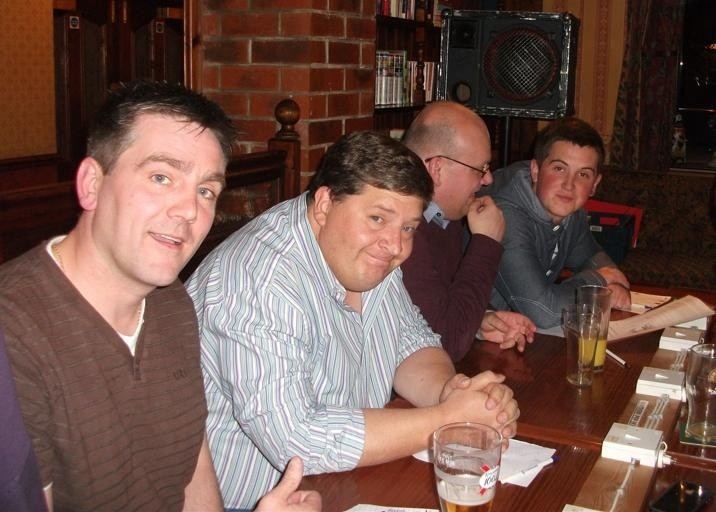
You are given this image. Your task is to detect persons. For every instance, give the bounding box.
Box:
[391,98,536,402]
[1,76,322,511]
[181,131,521,510]
[470,115,632,331]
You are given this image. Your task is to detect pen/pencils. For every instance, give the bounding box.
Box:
[606,348,627,366]
[500,456,560,485]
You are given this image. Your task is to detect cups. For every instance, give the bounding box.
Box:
[432,422,503,512]
[561,304,603,387]
[576,284,613,374]
[684,342,715,446]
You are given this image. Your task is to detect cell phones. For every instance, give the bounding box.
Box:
[649,479,716,512]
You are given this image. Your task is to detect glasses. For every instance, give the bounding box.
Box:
[424,154,491,178]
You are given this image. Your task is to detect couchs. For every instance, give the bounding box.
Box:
[590,165,716,288]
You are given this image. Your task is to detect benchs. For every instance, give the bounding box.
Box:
[0,99,302,285]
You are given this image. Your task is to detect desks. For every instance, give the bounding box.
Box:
[298,268,716,512]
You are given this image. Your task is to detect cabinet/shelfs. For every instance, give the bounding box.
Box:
[374,13,440,130]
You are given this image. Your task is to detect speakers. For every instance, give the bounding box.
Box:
[436,8,580,119]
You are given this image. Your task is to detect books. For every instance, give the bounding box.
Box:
[620,291,672,315]
[373,1,437,108]
